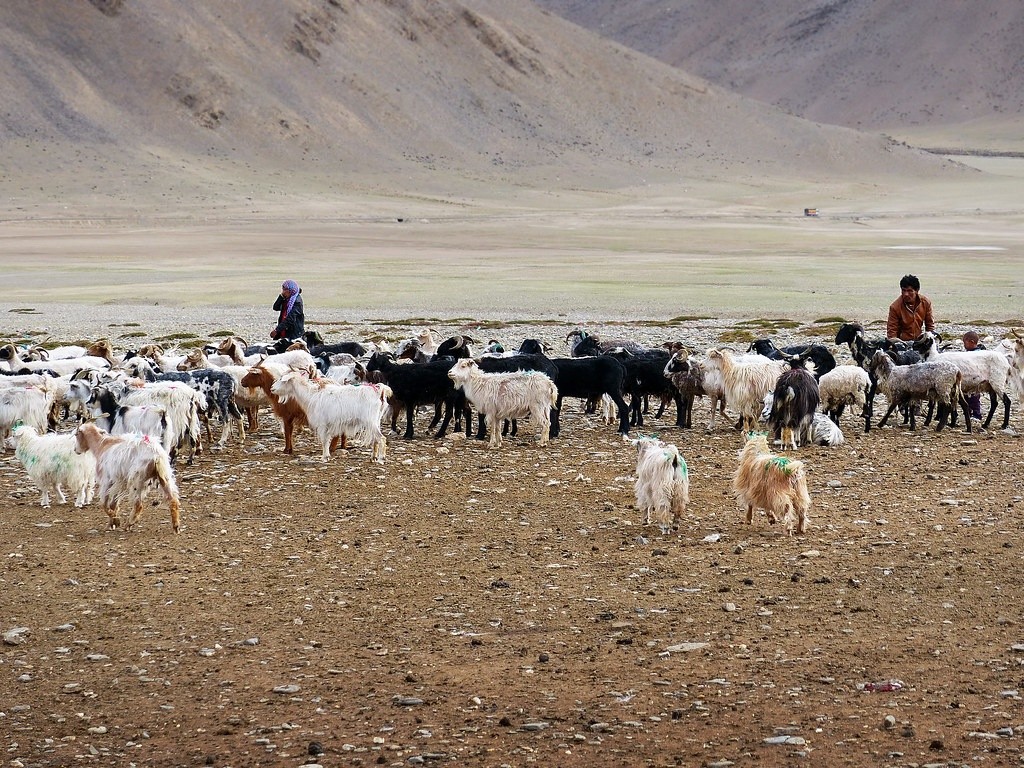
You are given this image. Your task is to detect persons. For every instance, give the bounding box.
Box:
[887,274,986,423]
[270,280,304,340]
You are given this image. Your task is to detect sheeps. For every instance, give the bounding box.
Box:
[269,370,394,465]
[3,424,96,509]
[71,411,181,536]
[622,433,690,535]
[731,428,814,538]
[0,321,1021,453]
